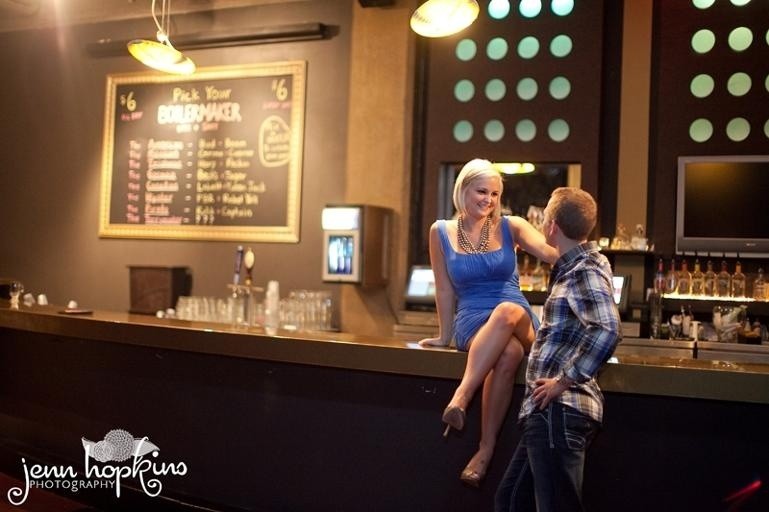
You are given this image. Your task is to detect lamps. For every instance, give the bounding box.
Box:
[126,0,196,76]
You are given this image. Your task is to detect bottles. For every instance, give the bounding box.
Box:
[176,294,239,324]
[713,302,764,345]
[264,278,279,326]
[679,305,693,337]
[279,287,333,331]
[518,254,555,291]
[651,254,767,303]
[265,326,277,337]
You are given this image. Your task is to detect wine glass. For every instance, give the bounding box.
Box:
[9,281,21,303]
[9,303,18,310]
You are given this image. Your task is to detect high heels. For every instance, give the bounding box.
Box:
[459,462,490,482]
[442,405,464,436]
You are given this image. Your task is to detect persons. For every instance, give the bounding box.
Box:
[492,185,623,511]
[419,156,562,490]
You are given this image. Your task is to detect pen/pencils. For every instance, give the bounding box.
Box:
[58,310,94,316]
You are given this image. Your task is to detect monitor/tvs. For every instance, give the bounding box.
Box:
[612,271,633,314]
[675,154,769,252]
[403,263,437,310]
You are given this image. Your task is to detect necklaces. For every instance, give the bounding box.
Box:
[456,214,492,254]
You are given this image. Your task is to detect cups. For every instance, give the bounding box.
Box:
[526,206,545,232]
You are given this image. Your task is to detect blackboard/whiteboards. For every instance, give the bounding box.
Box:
[98,58,306,243]
